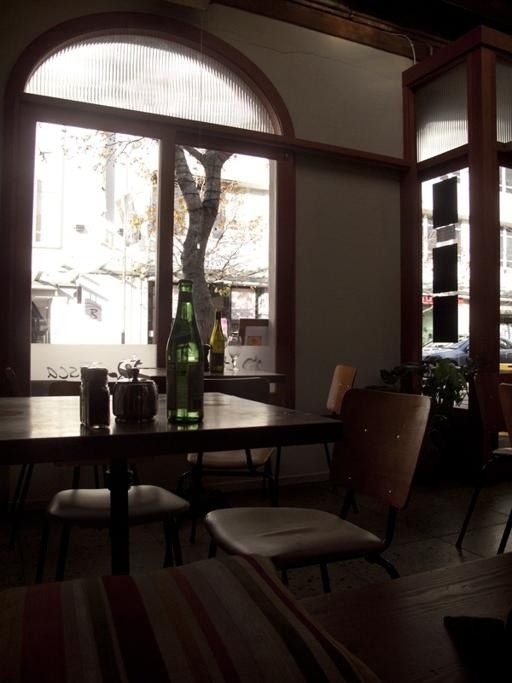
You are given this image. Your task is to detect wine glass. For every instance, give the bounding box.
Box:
[226,334,242,370]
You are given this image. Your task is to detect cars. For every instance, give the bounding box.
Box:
[422,333,511,380]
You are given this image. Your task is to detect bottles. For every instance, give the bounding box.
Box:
[208,310,224,374]
[79,367,109,429]
[164,276,205,426]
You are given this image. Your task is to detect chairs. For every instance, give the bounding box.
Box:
[206,389,436,592]
[2,367,48,585]
[494,381,512,558]
[46,375,191,578]
[272,366,360,512]
[455,372,510,551]
[176,378,277,542]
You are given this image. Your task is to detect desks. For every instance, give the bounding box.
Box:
[293,553,512,680]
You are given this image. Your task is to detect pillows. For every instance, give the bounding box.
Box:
[2,554,386,682]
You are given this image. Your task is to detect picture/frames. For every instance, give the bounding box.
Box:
[240,319,270,347]
